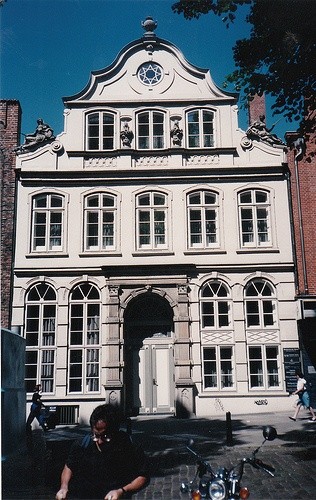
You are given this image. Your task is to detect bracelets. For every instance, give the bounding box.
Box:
[120,487,128,496]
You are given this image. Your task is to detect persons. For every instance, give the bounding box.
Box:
[26,384,49,432]
[288,371,316,422]
[251,115,284,146]
[55,404,150,500]
[21,118,53,144]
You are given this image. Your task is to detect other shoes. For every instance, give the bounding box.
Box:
[309,416,316,420]
[289,417,296,421]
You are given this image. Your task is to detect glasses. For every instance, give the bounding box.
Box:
[89,433,116,443]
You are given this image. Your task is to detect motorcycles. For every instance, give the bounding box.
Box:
[180,424,276,500]
